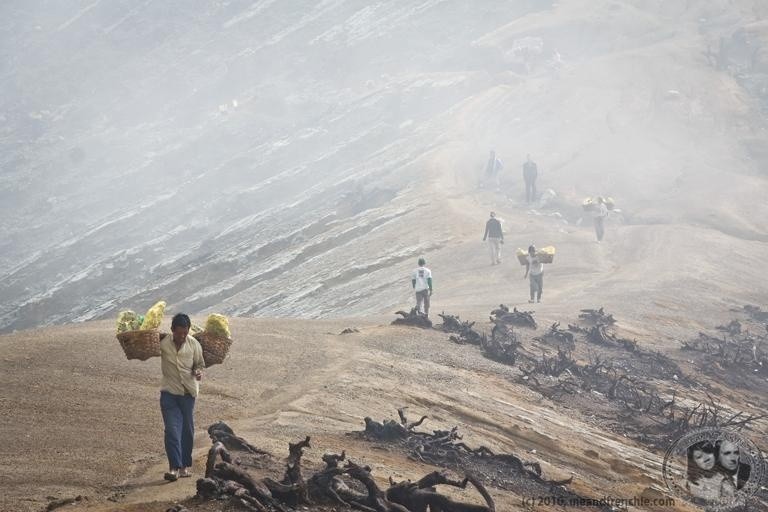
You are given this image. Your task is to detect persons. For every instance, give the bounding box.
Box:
[585,196,609,244]
[156,313,205,481]
[714,438,751,492]
[680,439,734,504]
[411,256,433,317]
[522,153,538,203]
[482,210,505,265]
[524,245,544,303]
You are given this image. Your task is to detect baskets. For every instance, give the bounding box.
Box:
[116,328,161,361]
[192,332,233,367]
[582,203,614,211]
[537,253,553,263]
[517,255,528,265]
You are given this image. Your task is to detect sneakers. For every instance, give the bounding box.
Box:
[165,466,193,482]
[528,298,540,303]
[492,258,502,266]
